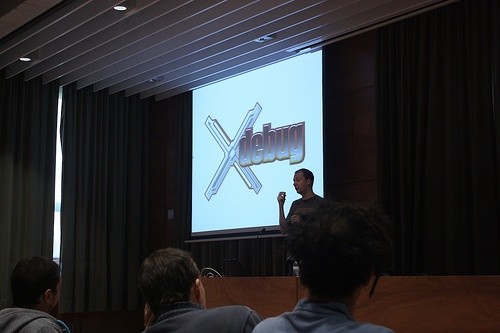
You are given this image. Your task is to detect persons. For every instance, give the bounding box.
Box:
[252,200,395,333]
[139,248,264,333]
[0,256,63,333]
[277,168,324,235]
